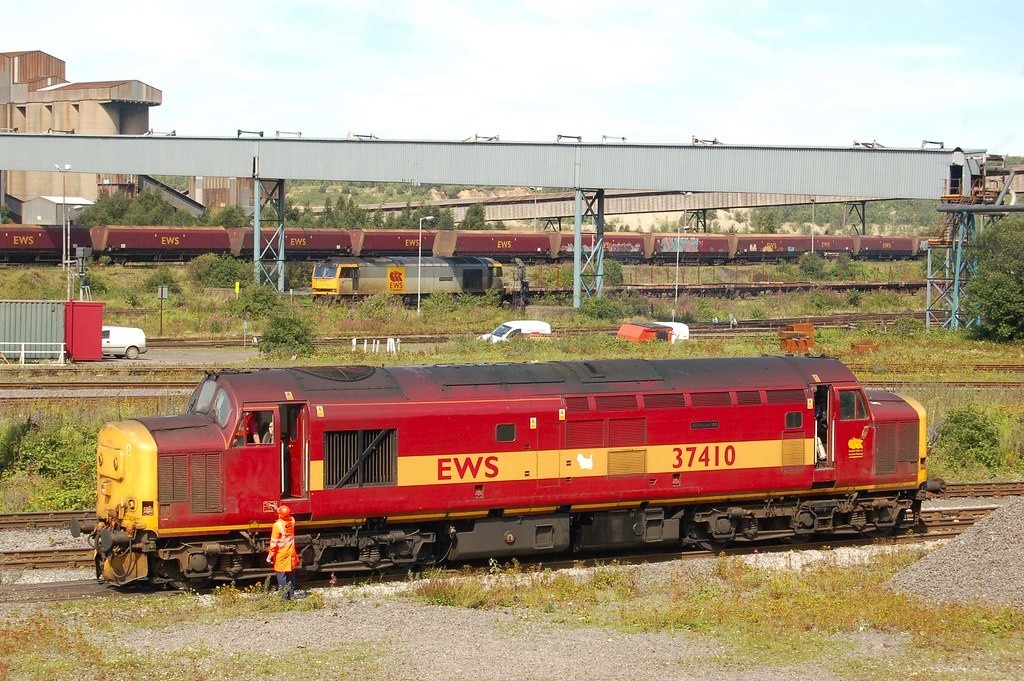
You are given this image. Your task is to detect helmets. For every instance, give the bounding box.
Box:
[277,505,290,520]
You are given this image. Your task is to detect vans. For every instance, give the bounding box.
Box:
[477,320,551,347]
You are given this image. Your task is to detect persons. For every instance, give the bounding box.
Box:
[247,412,260,444]
[265,502,298,602]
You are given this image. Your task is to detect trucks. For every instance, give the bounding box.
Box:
[616,322,691,345]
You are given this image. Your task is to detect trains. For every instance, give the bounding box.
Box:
[309,254,505,301]
[81,356,931,596]
[0,224,574,264]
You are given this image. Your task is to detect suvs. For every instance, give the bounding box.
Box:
[101,326,147,359]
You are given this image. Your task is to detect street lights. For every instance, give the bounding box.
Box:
[417,216,436,312]
[67,204,86,300]
[682,191,693,235]
[531,186,542,230]
[675,225,691,303]
[54,163,71,271]
